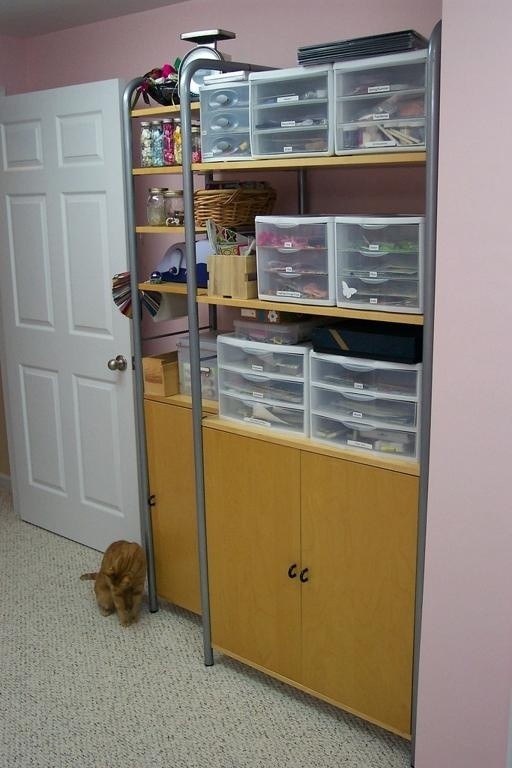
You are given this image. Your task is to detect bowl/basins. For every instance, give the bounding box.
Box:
[145,82,181,106]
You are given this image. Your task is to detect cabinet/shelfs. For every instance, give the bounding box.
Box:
[124,20,441,742]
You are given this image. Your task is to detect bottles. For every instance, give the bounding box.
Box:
[146,188,169,226]
[163,190,185,227]
[139,117,201,168]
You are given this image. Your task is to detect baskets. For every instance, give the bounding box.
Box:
[194,187,277,227]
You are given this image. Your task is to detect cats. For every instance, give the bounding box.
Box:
[79,541,147,626]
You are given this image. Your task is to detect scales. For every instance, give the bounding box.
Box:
[175,28,236,102]
